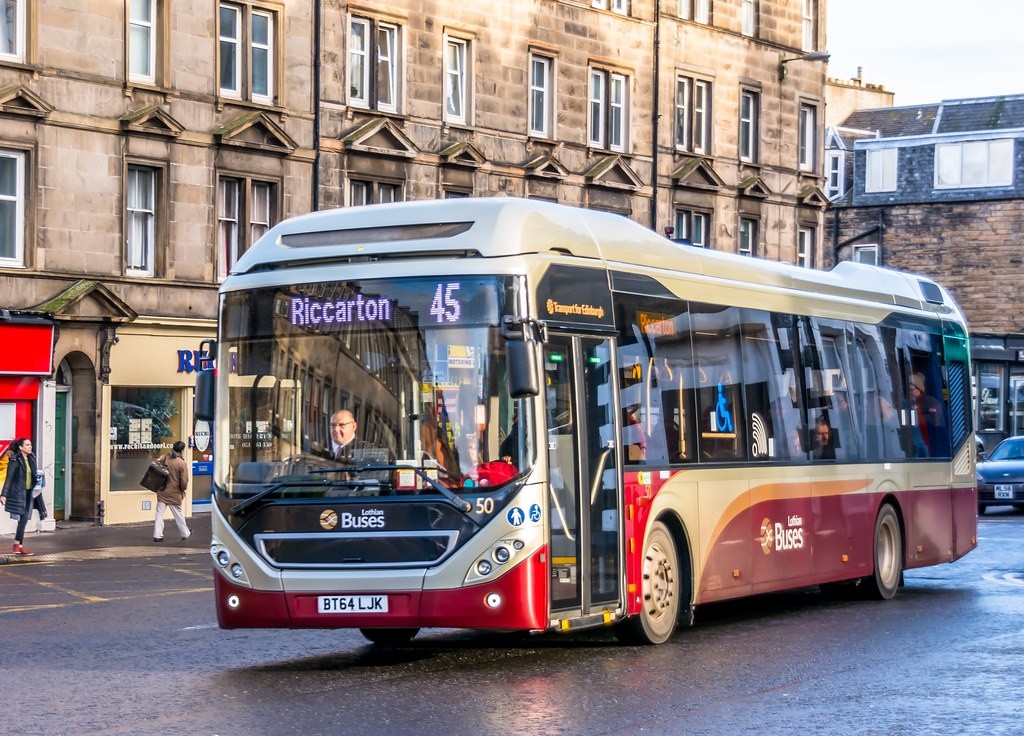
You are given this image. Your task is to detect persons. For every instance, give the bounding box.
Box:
[0,438,47,555]
[909,372,930,443]
[328,408,388,482]
[873,396,895,424]
[500,417,565,471]
[153,441,192,542]
[811,394,861,459]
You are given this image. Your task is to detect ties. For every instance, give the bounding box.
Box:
[336,446,342,460]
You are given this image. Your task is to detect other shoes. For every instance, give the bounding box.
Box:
[183,529,193,540]
[154,538,163,542]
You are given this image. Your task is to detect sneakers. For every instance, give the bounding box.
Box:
[17,549,34,557]
[11,544,22,554]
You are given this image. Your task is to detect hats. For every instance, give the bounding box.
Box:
[908,372,925,392]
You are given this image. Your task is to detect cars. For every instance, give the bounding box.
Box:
[976,436,1024,516]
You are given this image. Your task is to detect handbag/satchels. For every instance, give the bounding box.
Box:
[139,454,172,493]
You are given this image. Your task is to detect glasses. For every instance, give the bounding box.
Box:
[909,387,917,393]
[330,421,354,429]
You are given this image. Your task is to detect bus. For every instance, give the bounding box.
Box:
[202,196,978,653]
